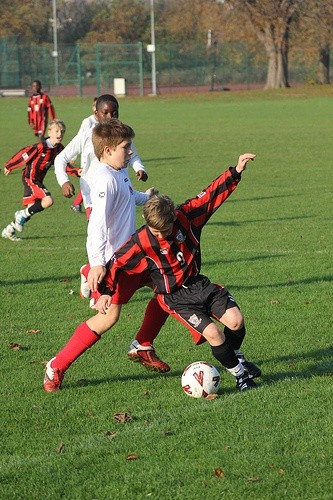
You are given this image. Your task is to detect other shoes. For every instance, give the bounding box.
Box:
[79,265,91,299]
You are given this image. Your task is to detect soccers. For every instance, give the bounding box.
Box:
[180,361,221,399]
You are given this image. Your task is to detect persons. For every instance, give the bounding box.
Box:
[99,154,262,391]
[28,80,57,141]
[1,119,83,241]
[71,97,98,213]
[43,116,170,392]
[54,94,149,308]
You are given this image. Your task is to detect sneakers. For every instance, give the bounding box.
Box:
[1,229,23,241]
[234,371,258,393]
[43,356,64,393]
[127,339,171,373]
[234,351,262,378]
[14,210,27,232]
[70,203,82,213]
